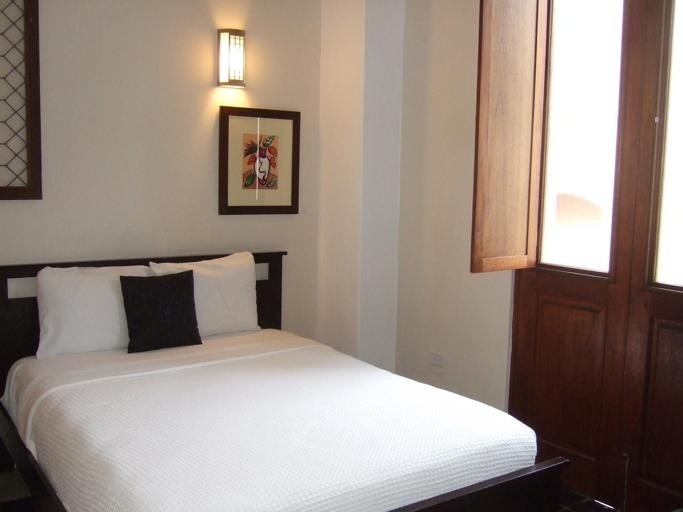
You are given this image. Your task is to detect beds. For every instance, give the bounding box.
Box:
[2,251,571,511]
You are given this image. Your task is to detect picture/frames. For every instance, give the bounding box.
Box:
[0,0,43,201]
[217,106,300,214]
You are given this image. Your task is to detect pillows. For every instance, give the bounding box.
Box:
[119,269,203,354]
[34,262,157,361]
[150,252,261,338]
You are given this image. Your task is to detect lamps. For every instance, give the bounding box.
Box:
[217,26,249,89]
